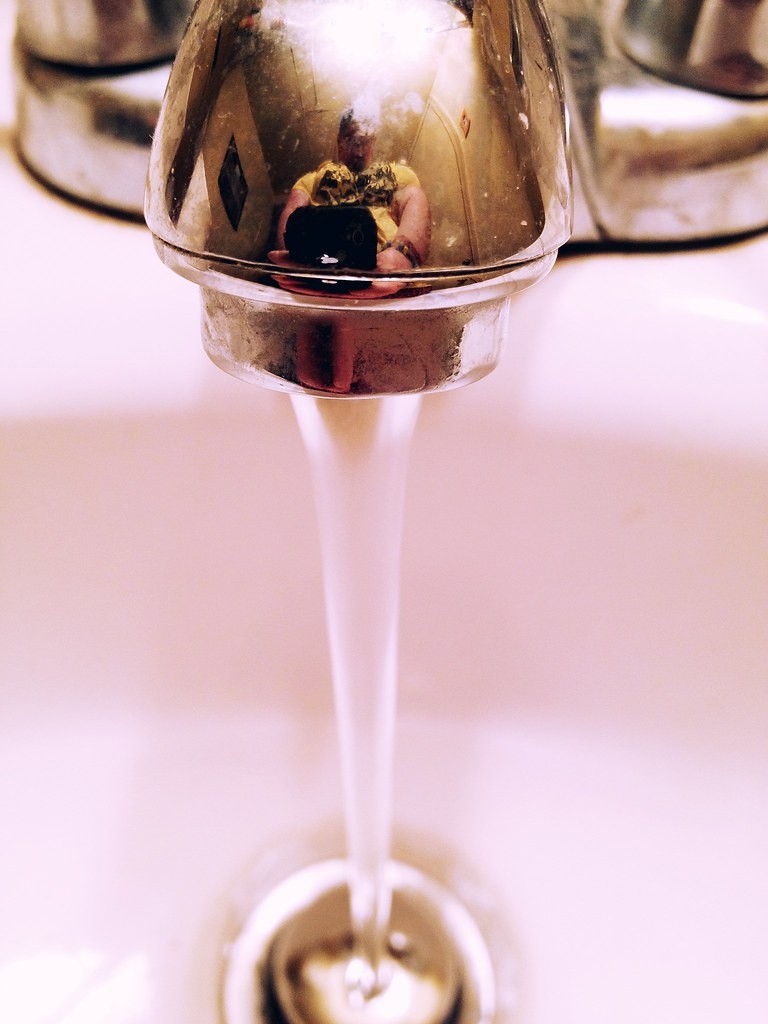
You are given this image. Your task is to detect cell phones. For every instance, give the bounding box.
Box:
[285,205,380,293]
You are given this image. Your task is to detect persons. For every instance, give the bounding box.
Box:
[267,107,431,299]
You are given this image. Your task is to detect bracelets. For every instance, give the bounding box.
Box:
[382,235,422,269]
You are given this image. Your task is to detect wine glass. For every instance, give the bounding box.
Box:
[142,0,573,1023]
